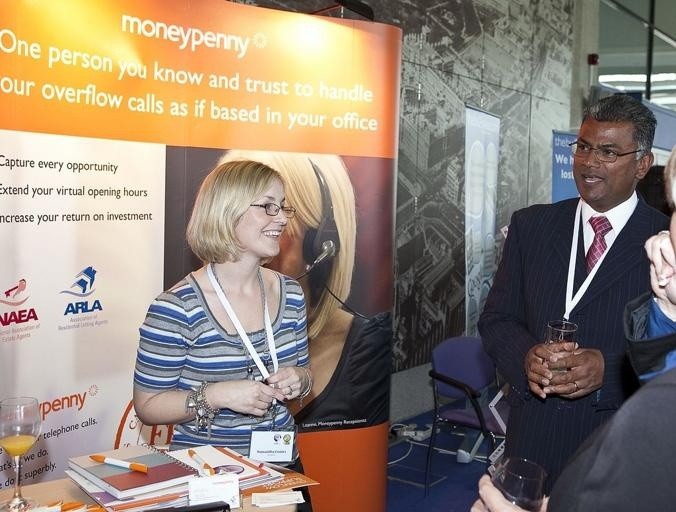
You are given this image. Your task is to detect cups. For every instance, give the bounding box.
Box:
[541,319,577,383]
[491,456,548,512]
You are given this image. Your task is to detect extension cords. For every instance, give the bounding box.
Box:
[401,426,440,441]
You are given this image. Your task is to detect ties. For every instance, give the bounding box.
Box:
[585,217,613,274]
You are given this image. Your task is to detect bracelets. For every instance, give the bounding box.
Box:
[184,379,219,439]
[297,367,312,408]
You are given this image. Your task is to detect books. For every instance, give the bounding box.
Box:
[65,442,320,512]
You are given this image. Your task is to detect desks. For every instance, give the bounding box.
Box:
[0,481,298,512]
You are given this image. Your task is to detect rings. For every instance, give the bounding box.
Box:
[659,230,669,235]
[573,382,579,392]
[288,386,293,396]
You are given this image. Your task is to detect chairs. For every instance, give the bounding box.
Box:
[424,336,509,498]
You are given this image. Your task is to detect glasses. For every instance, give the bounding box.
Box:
[249,202,297,219]
[567,140,642,164]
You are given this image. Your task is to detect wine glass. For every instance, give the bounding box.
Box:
[0,397,41,512]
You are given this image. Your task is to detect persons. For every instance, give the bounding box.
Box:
[477,96,670,497]
[469,143,676,512]
[218,150,391,432]
[132,160,313,512]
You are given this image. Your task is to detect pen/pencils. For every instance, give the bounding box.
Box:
[89,454,148,474]
[188,449,216,475]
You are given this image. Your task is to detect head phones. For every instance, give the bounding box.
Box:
[293,156,340,309]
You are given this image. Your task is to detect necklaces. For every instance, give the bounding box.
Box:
[212,263,271,419]
